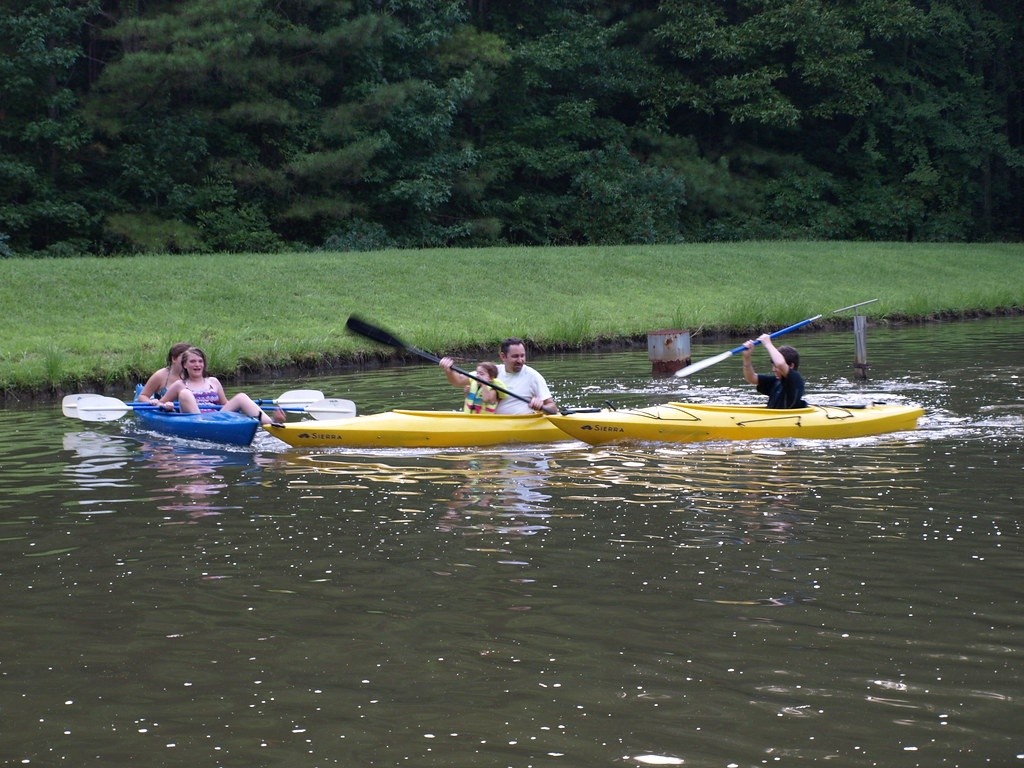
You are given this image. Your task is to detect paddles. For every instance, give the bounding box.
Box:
[343,314,560,418]
[675,296,883,378]
[76,396,359,423]
[61,389,325,414]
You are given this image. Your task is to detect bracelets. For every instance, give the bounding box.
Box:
[744,365,752,369]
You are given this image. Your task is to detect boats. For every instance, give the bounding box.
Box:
[260,407,579,447]
[544,398,923,445]
[133,384,259,446]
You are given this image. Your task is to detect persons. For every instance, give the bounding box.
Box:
[439,337,557,415]
[742,333,805,409]
[138,343,274,425]
